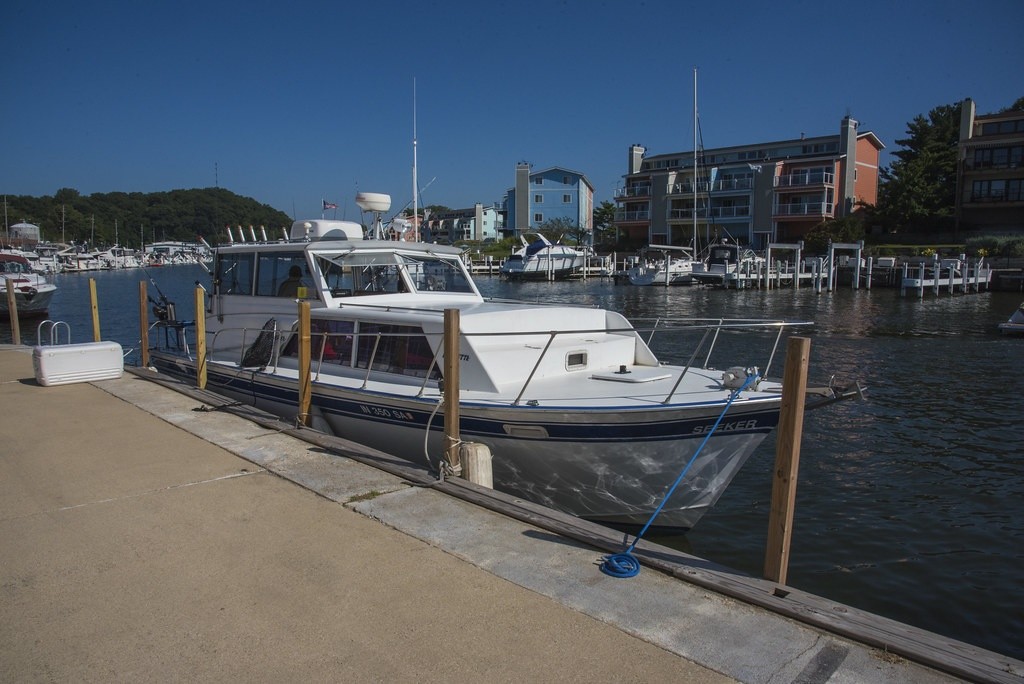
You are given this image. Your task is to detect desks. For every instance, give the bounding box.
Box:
[150,321,195,350]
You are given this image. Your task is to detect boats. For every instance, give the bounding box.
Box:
[0,246,57,317]
[998,303,1024,337]
[145,76,872,535]
[688,239,767,288]
[8,205,212,273]
[631,67,711,285]
[499,232,588,282]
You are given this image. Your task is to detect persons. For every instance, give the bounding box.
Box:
[278,265,307,297]
